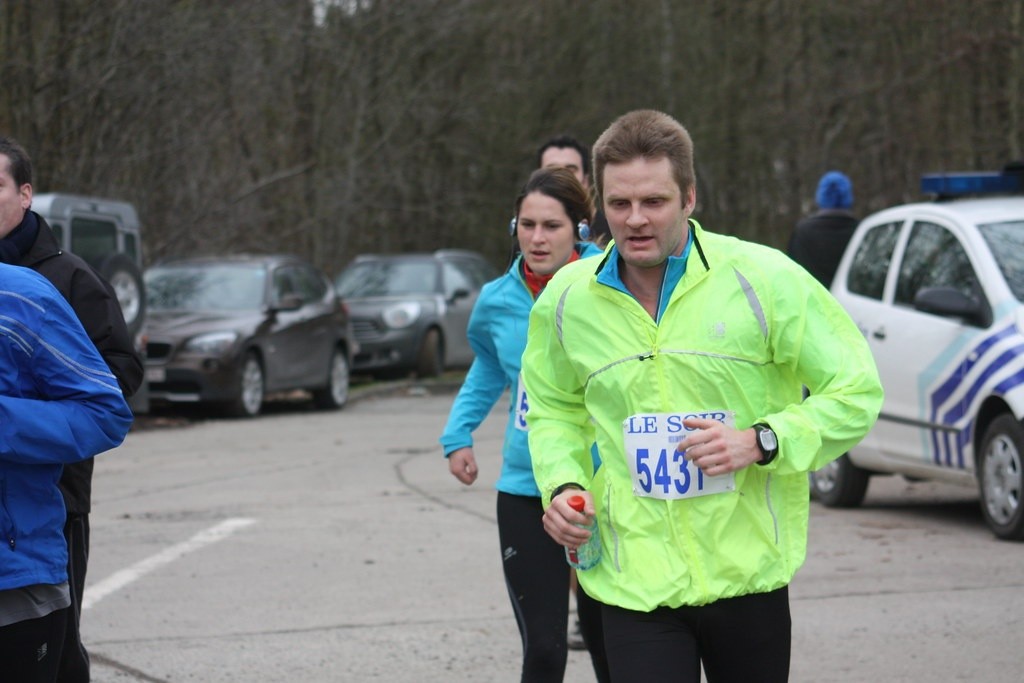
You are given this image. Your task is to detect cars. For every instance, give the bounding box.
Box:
[130,253,355,419]
[809,172,1024,544]
[332,250,506,387]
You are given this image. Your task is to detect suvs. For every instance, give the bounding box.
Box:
[28,190,151,417]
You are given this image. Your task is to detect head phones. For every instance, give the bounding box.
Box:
[509,215,590,241]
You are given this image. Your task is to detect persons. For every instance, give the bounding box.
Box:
[0,133,145,683]
[440,108,884,683]
[770,172,875,294]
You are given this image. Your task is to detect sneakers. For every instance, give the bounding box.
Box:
[567,610,587,651]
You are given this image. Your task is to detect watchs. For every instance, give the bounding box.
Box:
[750,423,779,466]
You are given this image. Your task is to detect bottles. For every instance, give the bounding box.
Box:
[565,497,603,572]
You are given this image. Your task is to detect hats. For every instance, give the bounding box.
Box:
[815,172,853,209]
